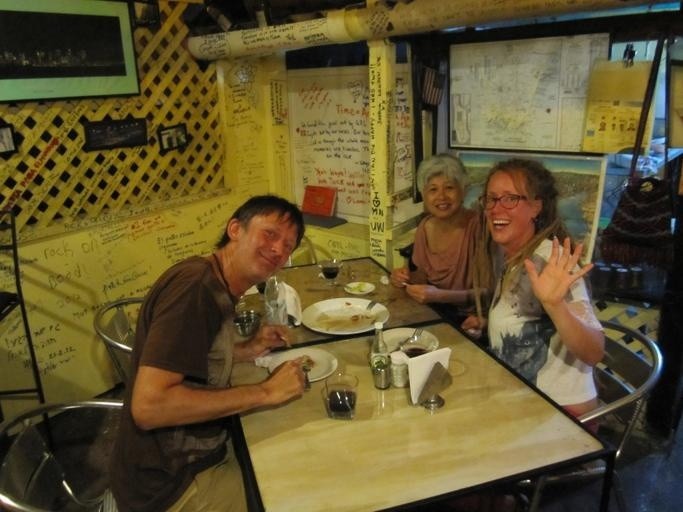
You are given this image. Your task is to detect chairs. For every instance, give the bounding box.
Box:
[93,294,152,390]
[1,396,212,512]
[495,318,666,512]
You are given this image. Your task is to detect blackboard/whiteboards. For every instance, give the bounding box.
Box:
[286,64,424,223]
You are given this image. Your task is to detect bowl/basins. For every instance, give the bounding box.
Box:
[379,328,443,355]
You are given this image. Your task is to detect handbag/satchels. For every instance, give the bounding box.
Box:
[601,176,673,250]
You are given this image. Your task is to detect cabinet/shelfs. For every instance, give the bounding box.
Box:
[0,206,47,441]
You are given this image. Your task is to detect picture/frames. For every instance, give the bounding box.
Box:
[0,0,141,104]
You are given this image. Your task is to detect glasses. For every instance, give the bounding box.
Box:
[477,194,543,210]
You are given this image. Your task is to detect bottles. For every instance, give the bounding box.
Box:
[366,321,409,388]
[257,276,291,328]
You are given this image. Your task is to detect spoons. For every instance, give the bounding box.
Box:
[302,365,313,391]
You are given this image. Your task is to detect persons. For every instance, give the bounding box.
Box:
[106,194,312,512]
[441,155,610,511]
[384,151,501,350]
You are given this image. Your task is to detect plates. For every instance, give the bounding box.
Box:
[298,297,391,336]
[269,350,339,383]
[344,280,376,296]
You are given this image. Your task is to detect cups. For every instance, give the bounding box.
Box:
[325,375,359,420]
[318,257,344,287]
[235,291,247,315]
[234,309,260,340]
